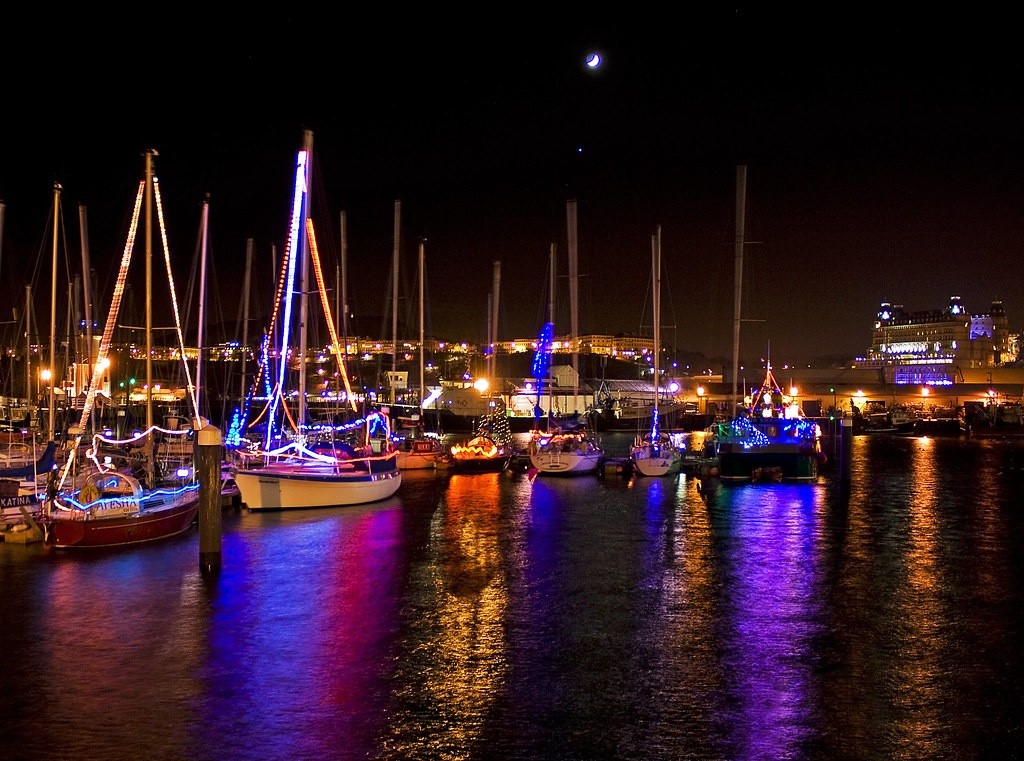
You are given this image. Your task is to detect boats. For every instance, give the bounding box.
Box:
[858,398,1024,436]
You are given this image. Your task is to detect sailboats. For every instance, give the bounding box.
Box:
[524,243,608,478]
[449,259,522,474]
[708,164,822,484]
[0,128,443,552]
[627,226,683,478]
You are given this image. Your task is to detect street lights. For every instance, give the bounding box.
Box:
[829,389,837,456]
[830,415,844,478]
